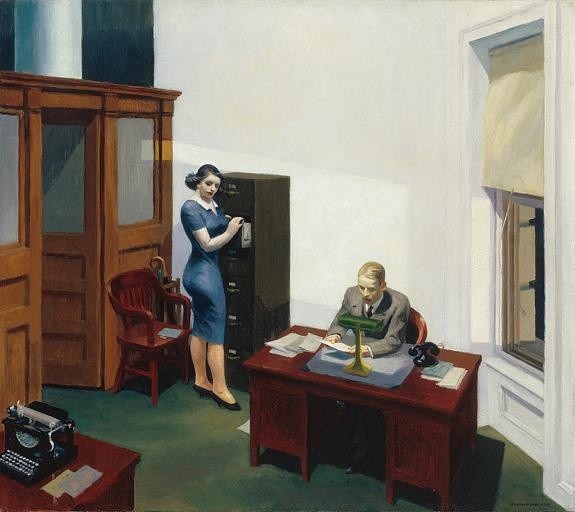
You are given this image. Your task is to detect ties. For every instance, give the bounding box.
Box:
[366,304,374,318]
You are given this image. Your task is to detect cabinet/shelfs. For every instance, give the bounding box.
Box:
[214,171,291,394]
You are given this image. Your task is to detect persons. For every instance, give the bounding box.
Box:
[179,162,243,411]
[320,259,412,476]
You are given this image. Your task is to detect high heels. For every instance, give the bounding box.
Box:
[207,390,241,411]
[192,382,214,399]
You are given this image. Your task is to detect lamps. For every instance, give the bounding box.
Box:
[339,314,384,378]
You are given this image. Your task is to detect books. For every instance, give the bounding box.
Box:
[265,330,353,360]
[421,360,469,390]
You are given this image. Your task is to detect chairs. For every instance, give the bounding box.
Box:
[107,268,192,407]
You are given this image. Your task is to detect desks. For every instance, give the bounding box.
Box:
[243,324,481,511]
[0,425,141,512]
